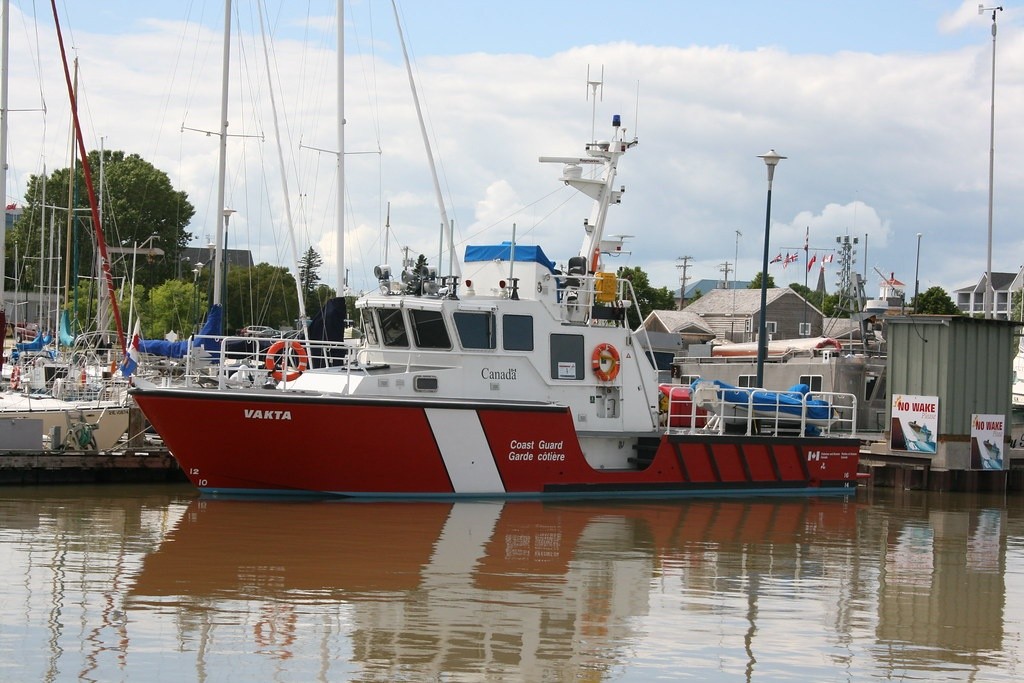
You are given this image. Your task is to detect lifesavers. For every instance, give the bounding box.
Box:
[266,340,308,379]
[815,338,841,357]
[592,342,619,380]
[10,367,20,389]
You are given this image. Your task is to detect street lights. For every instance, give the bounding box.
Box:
[194,262,205,334]
[977,3,1004,316]
[206,242,215,316]
[755,150,788,388]
[190,268,199,332]
[222,206,236,336]
[914,232,923,314]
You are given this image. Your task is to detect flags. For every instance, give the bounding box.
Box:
[808,253,817,272]
[789,252,799,263]
[770,252,782,264]
[120,317,141,380]
[6,203,18,210]
[821,255,827,272]
[804,234,809,251]
[782,252,791,269]
[827,253,834,263]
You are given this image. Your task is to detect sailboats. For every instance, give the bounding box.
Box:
[0,0,869,503]
